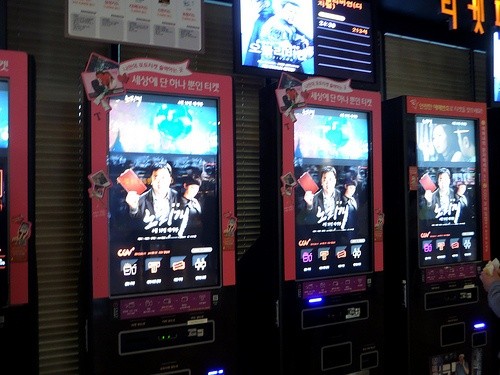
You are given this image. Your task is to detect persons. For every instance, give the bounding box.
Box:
[421,124,477,225]
[454,354,469,375]
[243,0,314,73]
[123,158,203,241]
[480,265,500,317]
[303,165,359,230]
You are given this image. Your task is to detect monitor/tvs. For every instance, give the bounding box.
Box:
[232,0,381,92]
[414,113,484,269]
[292,103,375,281]
[107,89,223,299]
